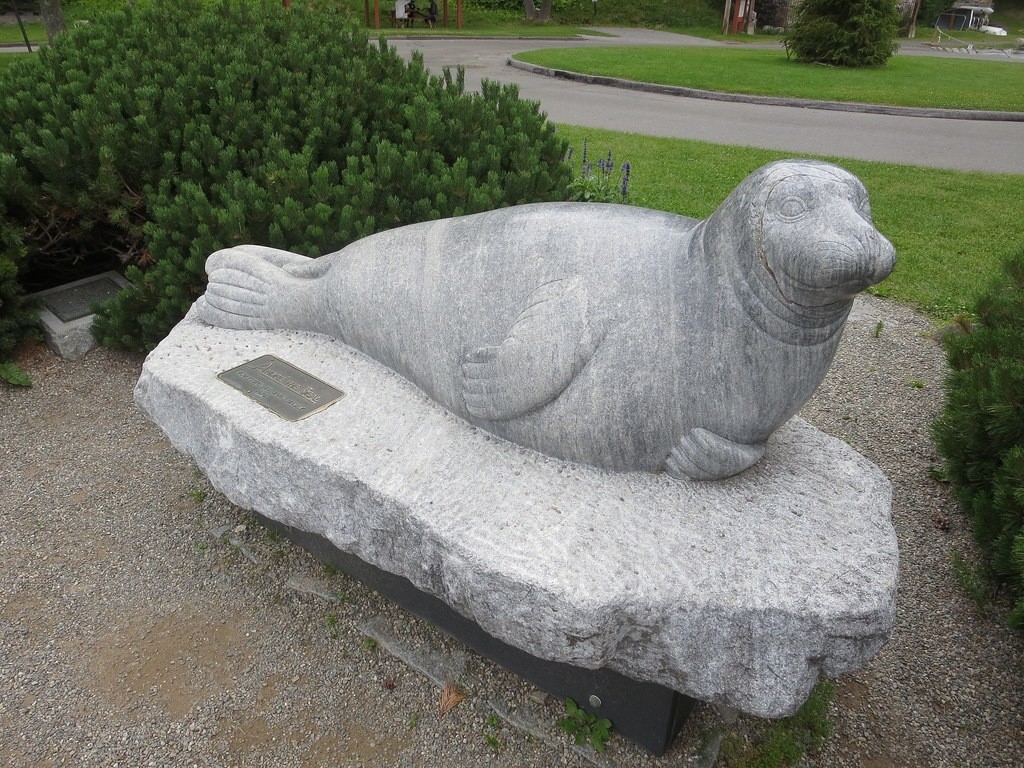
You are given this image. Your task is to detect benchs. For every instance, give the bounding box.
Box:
[388,15,438,29]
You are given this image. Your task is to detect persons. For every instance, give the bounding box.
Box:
[404,0,418,28]
[423,0,438,29]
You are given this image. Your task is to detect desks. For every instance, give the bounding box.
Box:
[388,7,423,27]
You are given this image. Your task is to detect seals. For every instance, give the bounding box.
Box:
[196,158,896,481]
